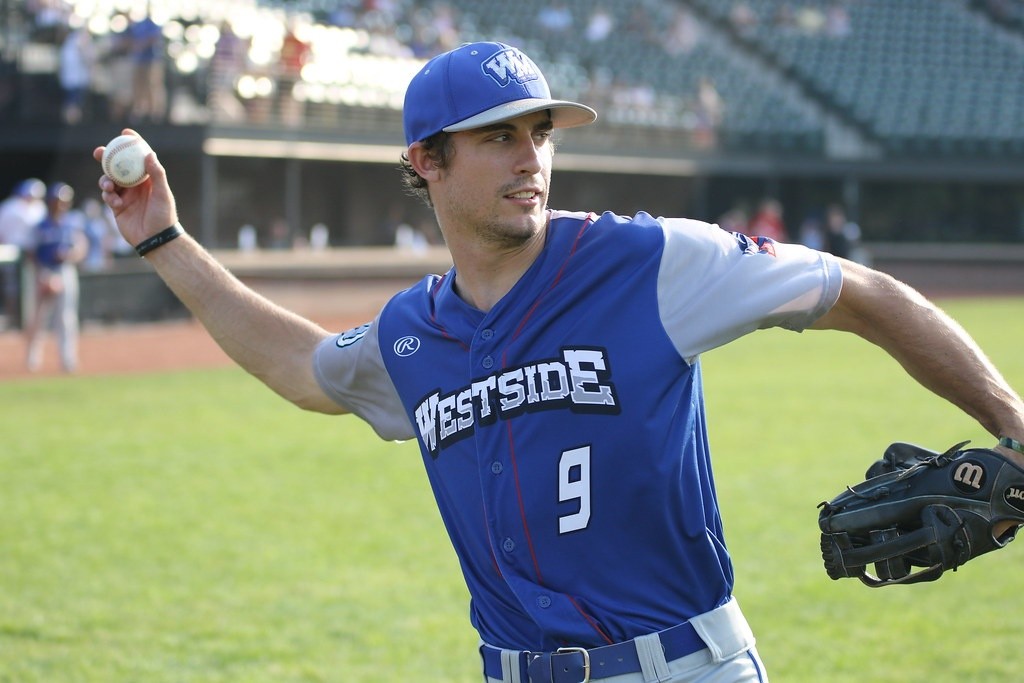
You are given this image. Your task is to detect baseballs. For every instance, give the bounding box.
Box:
[102,134,153,189]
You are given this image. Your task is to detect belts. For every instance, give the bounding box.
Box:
[480,620,707,683]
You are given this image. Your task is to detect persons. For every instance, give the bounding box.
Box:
[95,43,1024,683]
[0,0,864,375]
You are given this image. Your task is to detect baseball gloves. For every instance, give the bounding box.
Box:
[818,442,1024,588]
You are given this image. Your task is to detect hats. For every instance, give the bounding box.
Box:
[402,40,595,147]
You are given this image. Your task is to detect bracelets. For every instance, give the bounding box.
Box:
[134,222,185,259]
